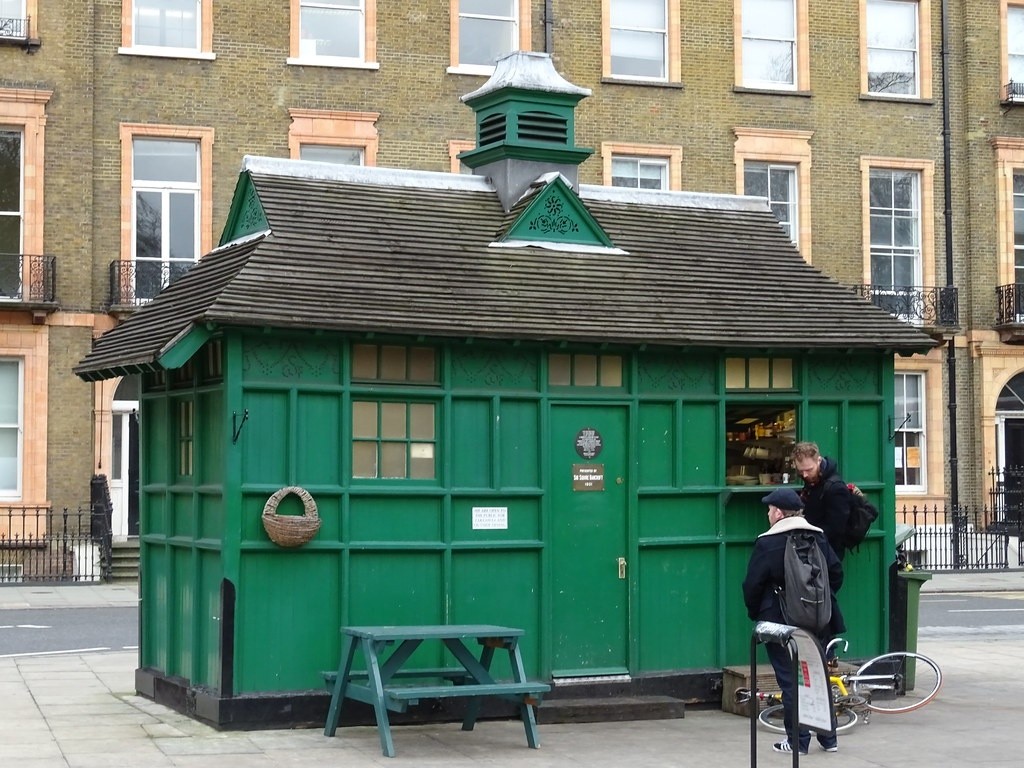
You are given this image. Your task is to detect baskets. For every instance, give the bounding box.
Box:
[260,485,322,547]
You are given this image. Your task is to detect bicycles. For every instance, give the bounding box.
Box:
[733,638,942,735]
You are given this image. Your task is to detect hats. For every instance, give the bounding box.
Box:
[762,487,805,510]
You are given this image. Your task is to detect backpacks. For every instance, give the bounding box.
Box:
[773,531,831,633]
[820,476,877,550]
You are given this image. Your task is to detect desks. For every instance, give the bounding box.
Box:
[323,625,540,758]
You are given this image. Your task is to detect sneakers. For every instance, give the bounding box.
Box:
[819,742,838,751]
[773,741,806,755]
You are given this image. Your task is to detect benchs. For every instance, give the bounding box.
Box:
[383,682,551,713]
[321,667,475,697]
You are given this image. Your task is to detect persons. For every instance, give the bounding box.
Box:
[791,441,850,668]
[742,488,844,755]
